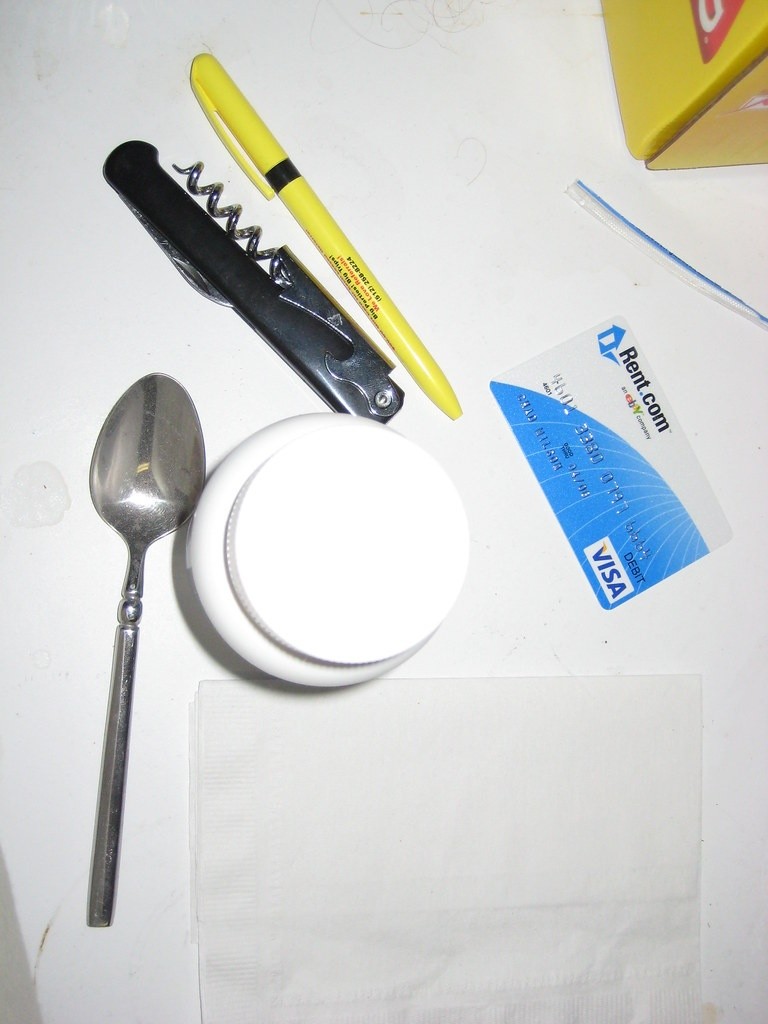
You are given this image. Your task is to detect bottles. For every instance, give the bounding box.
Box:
[185,415,460,688]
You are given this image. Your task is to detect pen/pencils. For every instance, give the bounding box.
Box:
[190,54,466,423]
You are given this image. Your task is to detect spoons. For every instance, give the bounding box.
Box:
[86,372,206,927]
